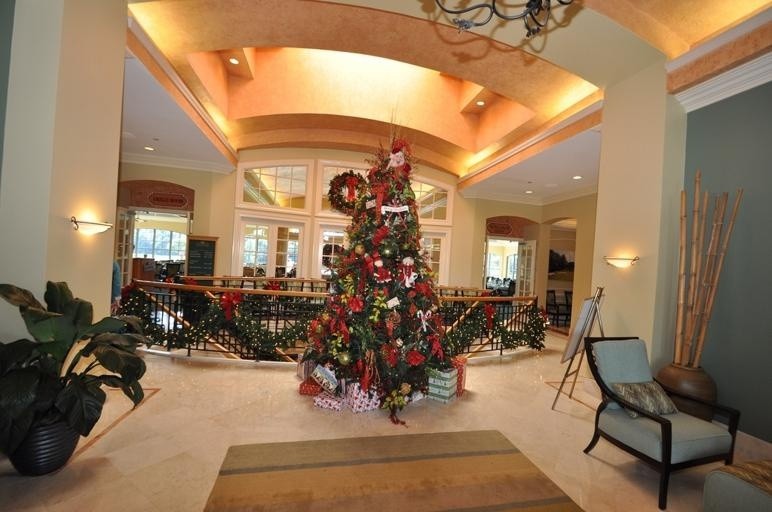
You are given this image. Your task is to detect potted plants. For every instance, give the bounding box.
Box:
[0,279,154,477]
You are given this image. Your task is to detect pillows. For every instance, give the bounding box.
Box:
[611,377,676,420]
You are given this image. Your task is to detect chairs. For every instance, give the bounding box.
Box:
[584,336,742,510]
[545,288,573,327]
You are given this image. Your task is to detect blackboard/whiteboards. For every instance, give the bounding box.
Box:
[184,235,220,286]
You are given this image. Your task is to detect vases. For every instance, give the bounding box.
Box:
[651,361,720,426]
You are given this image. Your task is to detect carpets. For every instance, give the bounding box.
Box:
[202,427,589,512]
